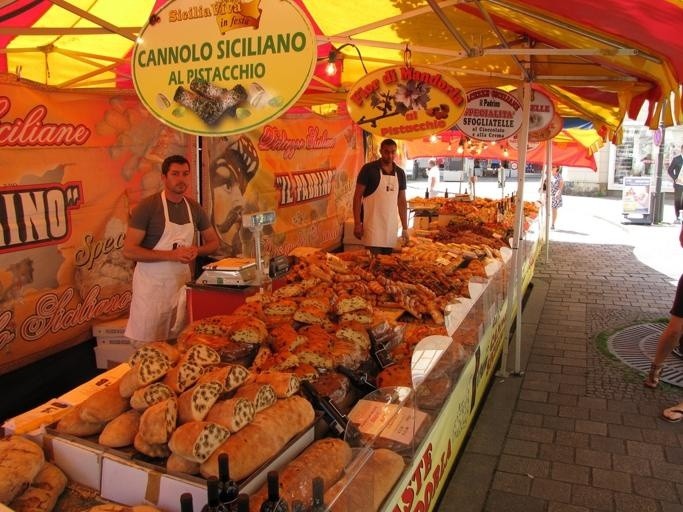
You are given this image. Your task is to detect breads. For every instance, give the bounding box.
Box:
[1,191,539,512]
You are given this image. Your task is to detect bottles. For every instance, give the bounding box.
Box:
[444,187,449,198]
[496,191,518,225]
[299,379,362,447]
[365,327,394,372]
[177,452,334,512]
[465,188,468,195]
[425,187,429,199]
[335,365,379,398]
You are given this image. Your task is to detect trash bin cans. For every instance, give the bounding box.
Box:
[650,192,664,224]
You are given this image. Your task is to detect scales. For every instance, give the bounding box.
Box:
[195,211,276,288]
[455,176,476,202]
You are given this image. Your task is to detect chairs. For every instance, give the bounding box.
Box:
[46,352,84,386]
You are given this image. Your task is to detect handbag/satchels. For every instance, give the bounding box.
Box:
[536,174,548,193]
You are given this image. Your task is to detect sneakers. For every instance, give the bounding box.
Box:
[642,361,664,388]
[550,224,556,230]
[672,344,683,357]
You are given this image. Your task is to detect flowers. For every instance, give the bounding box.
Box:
[395,80,431,111]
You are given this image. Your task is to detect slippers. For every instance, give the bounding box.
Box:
[659,400,683,424]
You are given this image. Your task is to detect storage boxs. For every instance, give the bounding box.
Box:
[344,245,361,252]
[100,425,315,512]
[45,420,104,491]
[93,345,133,369]
[343,218,362,245]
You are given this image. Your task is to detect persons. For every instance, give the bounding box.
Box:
[541,165,563,231]
[666,145,683,224]
[352,138,410,254]
[640,227,682,424]
[121,155,221,357]
[424,159,438,198]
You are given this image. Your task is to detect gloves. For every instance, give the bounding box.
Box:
[400,229,409,244]
